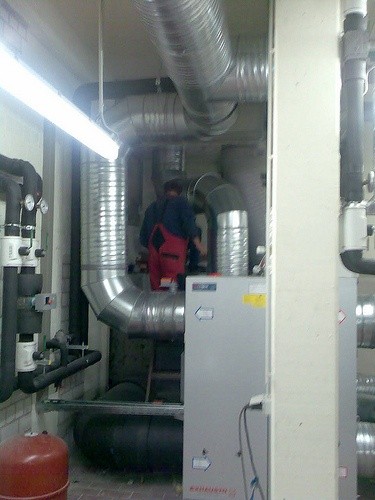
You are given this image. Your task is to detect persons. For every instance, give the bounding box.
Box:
[137,175,208,292]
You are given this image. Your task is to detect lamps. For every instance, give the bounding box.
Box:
[0,36,129,166]
